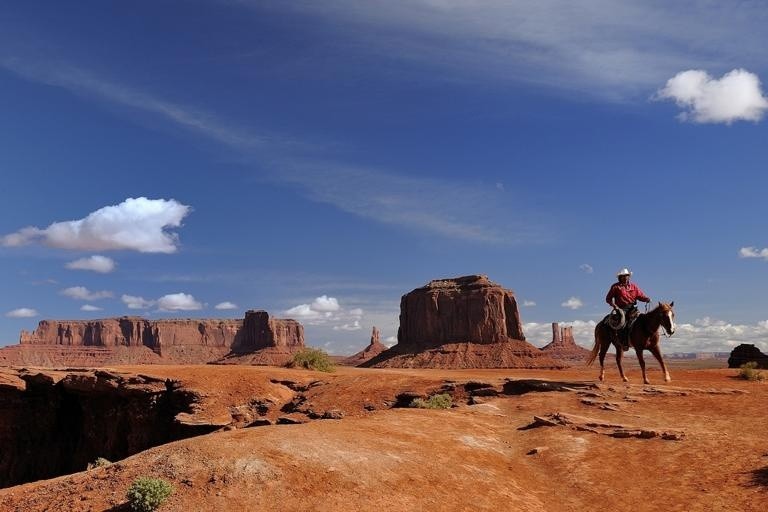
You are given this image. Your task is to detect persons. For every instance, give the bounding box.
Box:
[604,269,652,350]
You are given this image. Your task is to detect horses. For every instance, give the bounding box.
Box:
[584,300,675,384]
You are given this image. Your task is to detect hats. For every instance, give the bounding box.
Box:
[617,269,631,275]
